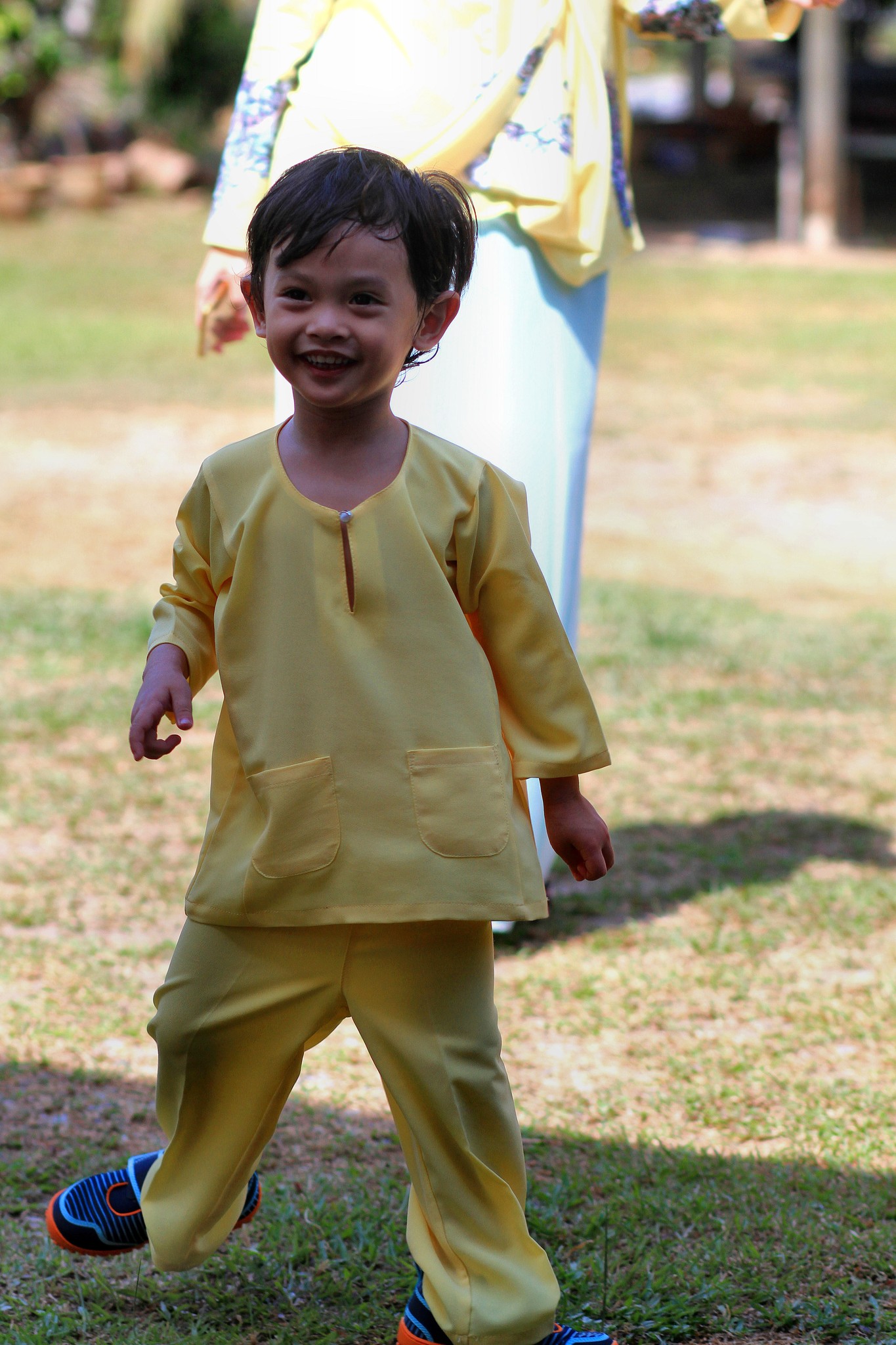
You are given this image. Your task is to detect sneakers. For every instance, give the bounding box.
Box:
[44,1149,259,1258]
[395,1261,616,1345]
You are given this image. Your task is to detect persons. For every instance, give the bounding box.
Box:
[42,143,630,1345]
[196,0,846,940]
